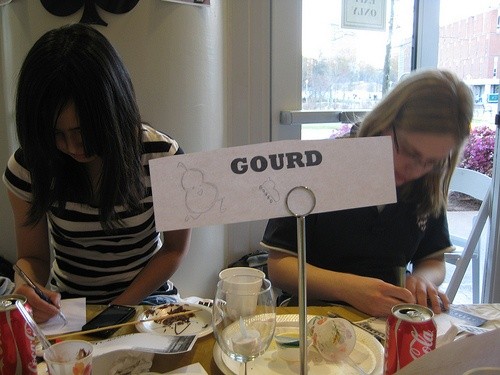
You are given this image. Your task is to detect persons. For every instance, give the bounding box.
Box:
[3,23,191,324]
[259,68,474,320]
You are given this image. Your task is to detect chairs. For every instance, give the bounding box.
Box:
[441,168,493,305]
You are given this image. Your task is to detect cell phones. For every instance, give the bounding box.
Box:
[82,304,135,339]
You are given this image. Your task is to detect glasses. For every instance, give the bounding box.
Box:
[392,122,451,170]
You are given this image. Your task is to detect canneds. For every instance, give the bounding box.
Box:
[0,294,38,375]
[382,303,437,375]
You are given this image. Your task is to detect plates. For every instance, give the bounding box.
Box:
[213,313,384,375]
[135,302,224,339]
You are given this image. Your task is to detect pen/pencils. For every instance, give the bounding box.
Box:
[399,265,406,288]
[13,264,66,322]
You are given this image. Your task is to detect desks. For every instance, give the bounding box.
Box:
[43,305,386,375]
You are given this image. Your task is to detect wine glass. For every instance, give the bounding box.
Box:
[211,275,276,375]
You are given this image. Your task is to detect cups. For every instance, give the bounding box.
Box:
[219,267,265,322]
[42,340,94,375]
[306,316,356,362]
[275,332,313,375]
[463,367,500,375]
